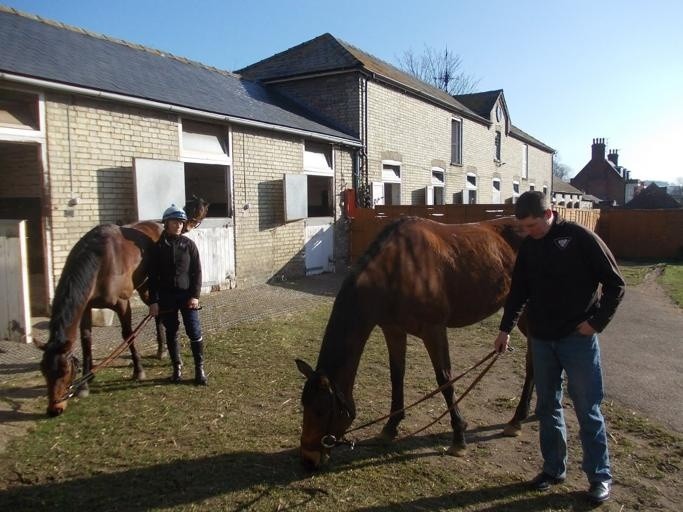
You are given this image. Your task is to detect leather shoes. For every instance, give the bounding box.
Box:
[527,474,566,491]
[586,478,612,503]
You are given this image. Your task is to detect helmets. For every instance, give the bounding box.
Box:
[161,204,187,223]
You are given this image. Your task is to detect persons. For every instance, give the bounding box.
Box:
[493,191,626,503]
[145,203,209,387]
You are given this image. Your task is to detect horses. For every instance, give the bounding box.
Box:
[181,192,212,234]
[32,220,165,417]
[295,216,543,468]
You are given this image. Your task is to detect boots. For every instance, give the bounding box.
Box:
[167,341,184,383]
[191,340,208,386]
